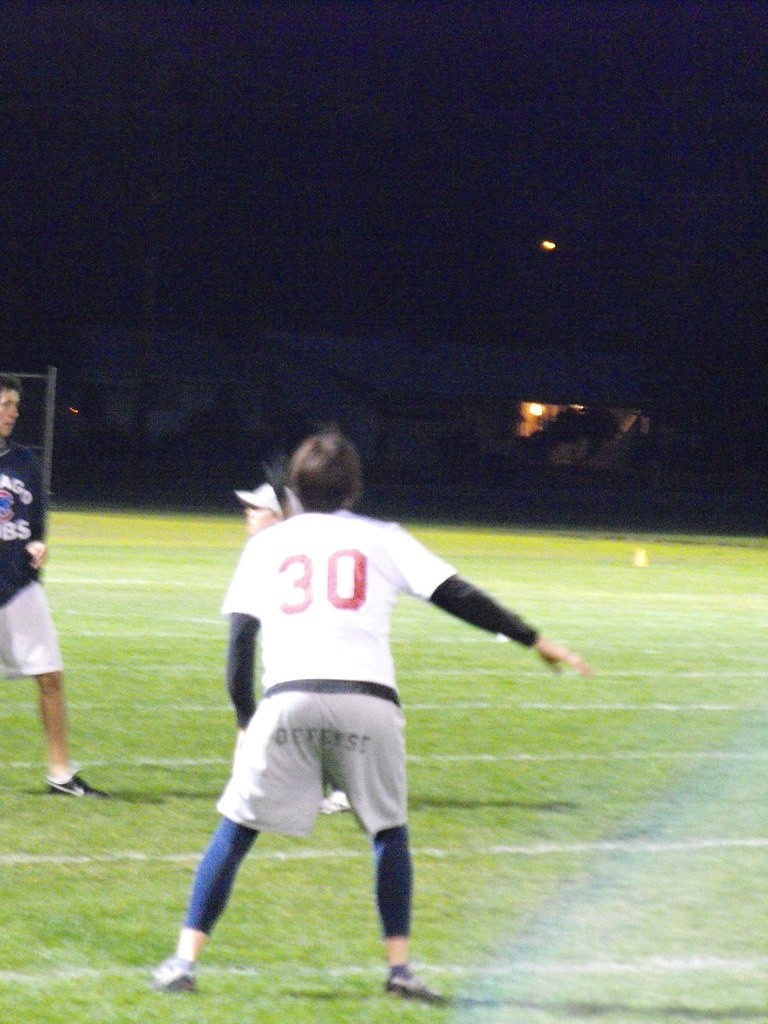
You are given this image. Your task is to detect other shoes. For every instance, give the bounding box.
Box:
[321,790,353,814]
[387,971,447,1003]
[154,959,197,992]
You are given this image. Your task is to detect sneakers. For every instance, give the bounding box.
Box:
[47,767,106,797]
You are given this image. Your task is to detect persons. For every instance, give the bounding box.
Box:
[232,479,351,813]
[150,435,595,1003]
[0,372,112,800]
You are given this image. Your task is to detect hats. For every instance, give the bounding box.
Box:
[234,482,304,520]
